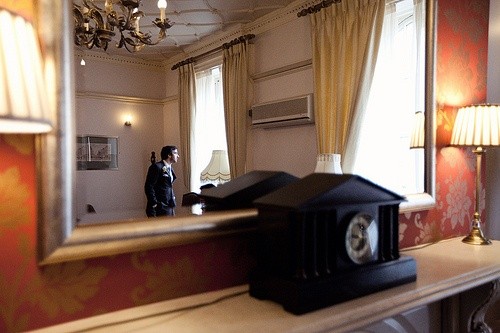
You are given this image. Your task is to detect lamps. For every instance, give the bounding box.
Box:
[200,150,231,187]
[0,6,53,134]
[72,0,175,53]
[409,111,425,149]
[314,153,343,175]
[449,104,500,245]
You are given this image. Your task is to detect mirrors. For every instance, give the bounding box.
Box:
[33,0,437,266]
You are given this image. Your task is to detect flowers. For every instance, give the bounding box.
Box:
[162,166,168,173]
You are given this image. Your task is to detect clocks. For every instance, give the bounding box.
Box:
[249,172,416,316]
[201,170,300,213]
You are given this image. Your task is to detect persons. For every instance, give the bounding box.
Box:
[144,145,180,219]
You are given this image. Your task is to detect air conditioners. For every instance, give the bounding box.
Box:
[252,93,315,129]
